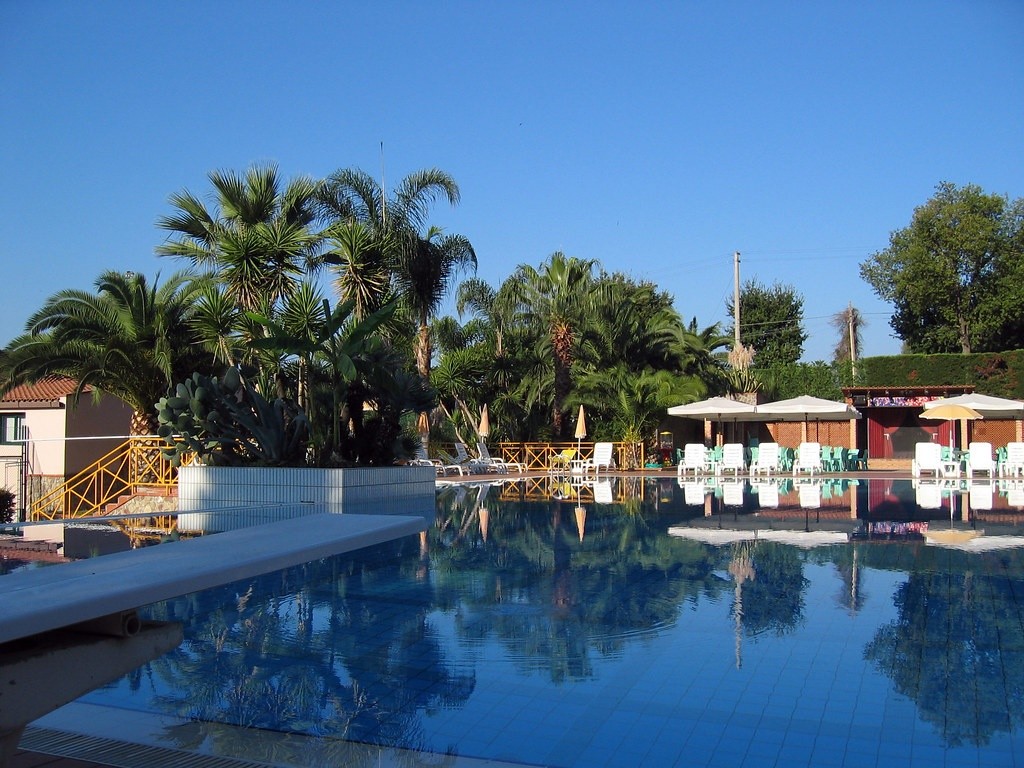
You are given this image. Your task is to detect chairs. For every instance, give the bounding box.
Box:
[436,449,474,476]
[585,442,616,475]
[476,443,528,474]
[675,442,1024,479]
[548,450,576,474]
[456,443,500,476]
[410,458,464,476]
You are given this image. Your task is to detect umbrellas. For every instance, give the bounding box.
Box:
[924,391,1024,420]
[668,396,756,445]
[416,411,430,438]
[574,486,587,541]
[420,530,427,555]
[755,393,863,444]
[665,508,864,549]
[478,403,491,443]
[924,508,1024,554]
[478,499,488,543]
[918,489,986,546]
[918,406,984,462]
[574,404,588,459]
